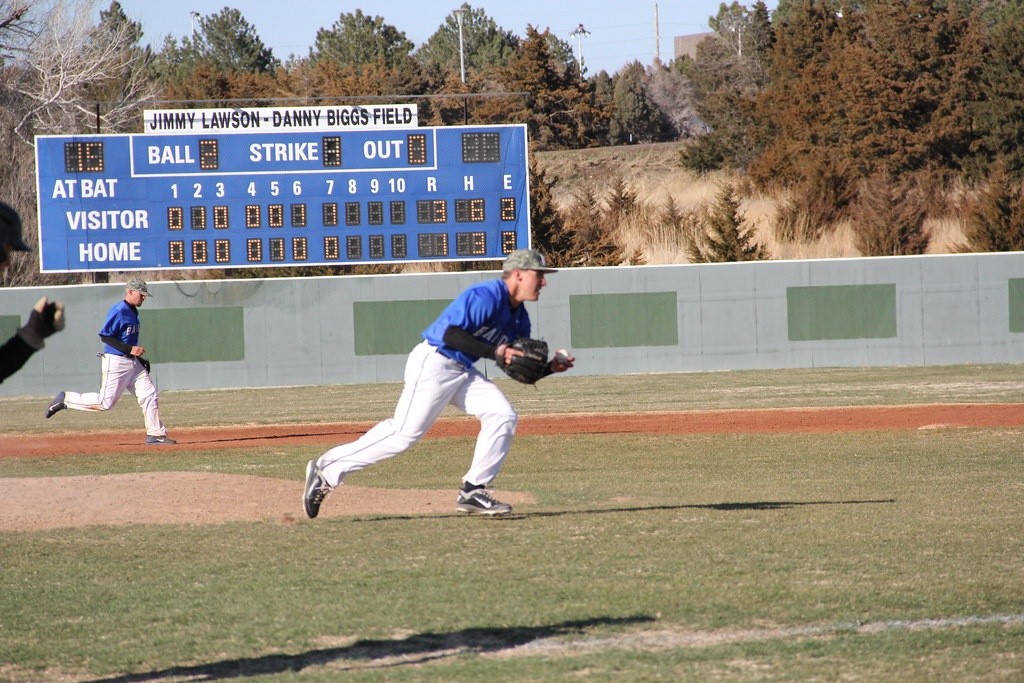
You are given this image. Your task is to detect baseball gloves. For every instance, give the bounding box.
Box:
[494,335,549,390]
[138,357,150,374]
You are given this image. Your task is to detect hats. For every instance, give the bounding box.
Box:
[0,203,33,251]
[502,249,558,273]
[126,278,153,298]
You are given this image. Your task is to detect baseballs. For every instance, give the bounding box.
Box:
[558,348,570,363]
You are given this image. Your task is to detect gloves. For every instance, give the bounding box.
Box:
[16,296,64,349]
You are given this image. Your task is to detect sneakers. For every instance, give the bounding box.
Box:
[146,436,177,445]
[303,460,332,519]
[45,392,66,418]
[456,490,512,514]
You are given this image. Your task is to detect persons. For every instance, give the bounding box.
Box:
[46,279,176,445]
[302,250,576,517]
[0,202,67,390]
